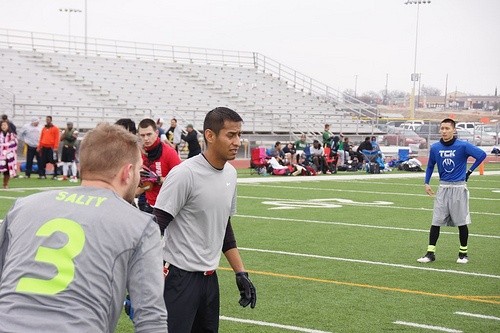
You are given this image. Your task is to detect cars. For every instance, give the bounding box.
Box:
[382,129,427,147]
[377,119,500,149]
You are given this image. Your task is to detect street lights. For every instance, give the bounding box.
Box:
[59,8,83,55]
[404,0,432,123]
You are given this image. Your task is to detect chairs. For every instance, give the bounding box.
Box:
[393,149,410,171]
[360,150,386,174]
[250,147,270,176]
[324,148,338,174]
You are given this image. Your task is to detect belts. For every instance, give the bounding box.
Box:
[204,270,215,275]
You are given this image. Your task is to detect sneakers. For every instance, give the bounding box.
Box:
[456,251,468,263]
[417,251,435,263]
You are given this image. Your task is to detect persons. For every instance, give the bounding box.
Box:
[0,123,169,333]
[0,113,202,281]
[416,118,487,264]
[125,106,256,333]
[251,124,425,176]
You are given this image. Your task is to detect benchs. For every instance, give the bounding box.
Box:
[0,48,385,134]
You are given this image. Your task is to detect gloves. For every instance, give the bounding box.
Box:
[140,166,161,186]
[236,271,256,309]
[465,172,470,182]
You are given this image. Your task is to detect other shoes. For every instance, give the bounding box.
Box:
[3,185,8,189]
[52,177,57,179]
[57,176,68,180]
[69,176,78,183]
[40,175,46,179]
[26,176,30,178]
